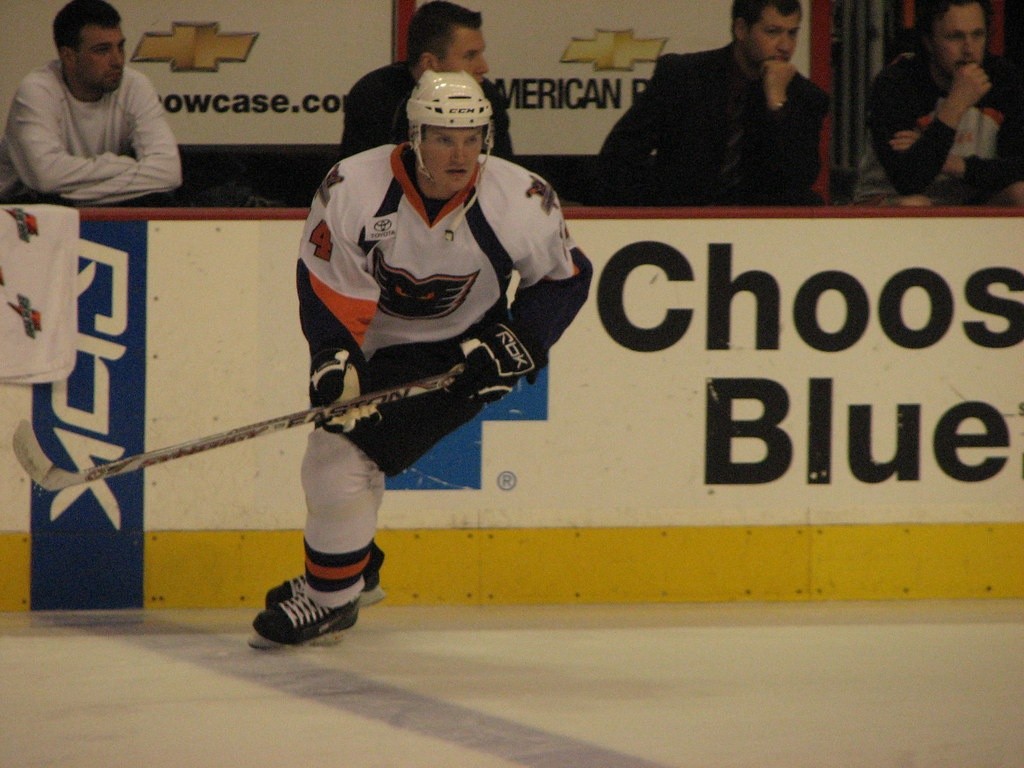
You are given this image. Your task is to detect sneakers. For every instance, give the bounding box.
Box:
[240,575,365,649]
[266,550,385,606]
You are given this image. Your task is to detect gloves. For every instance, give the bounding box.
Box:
[439,321,546,410]
[309,348,382,433]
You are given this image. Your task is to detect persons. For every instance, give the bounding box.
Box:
[0,1,185,206]
[852,1,1024,207]
[246,71,590,652]
[588,1,831,207]
[340,1,519,159]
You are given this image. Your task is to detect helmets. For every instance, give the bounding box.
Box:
[405,68,493,149]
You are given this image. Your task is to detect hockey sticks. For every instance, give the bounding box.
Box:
[11,366,463,494]
